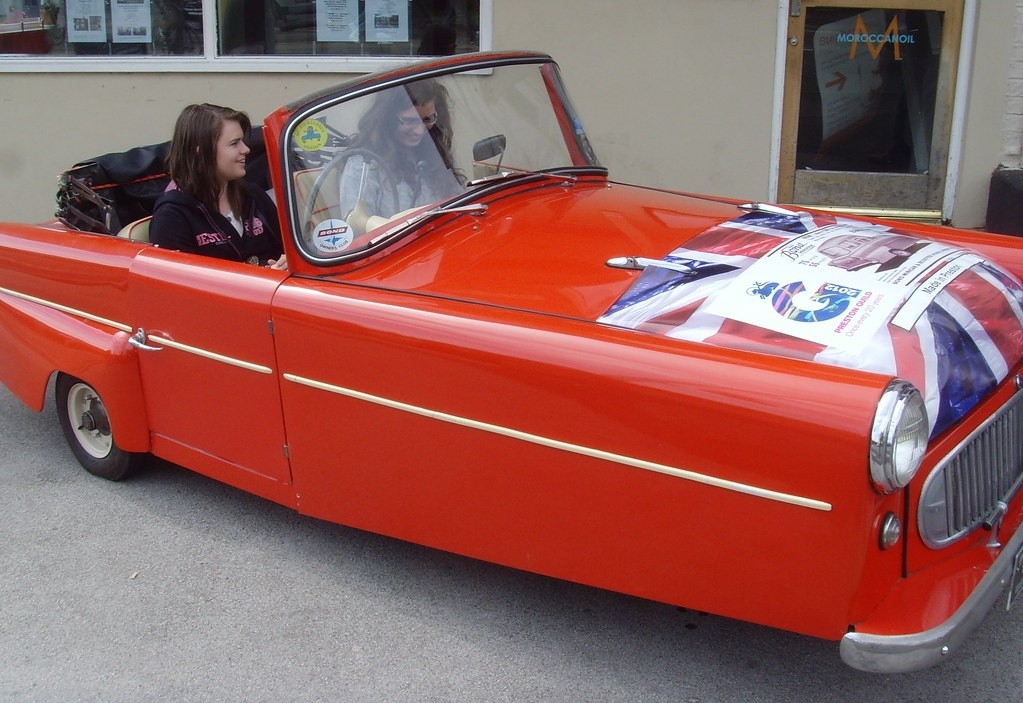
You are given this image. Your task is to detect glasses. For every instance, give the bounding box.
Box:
[395,110,439,127]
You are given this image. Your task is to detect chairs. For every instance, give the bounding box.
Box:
[295,165,342,252]
[117,215,151,244]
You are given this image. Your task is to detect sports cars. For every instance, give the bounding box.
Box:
[1,51,1023,675]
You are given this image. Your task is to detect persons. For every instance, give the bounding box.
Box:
[148,103,293,271]
[339,80,466,236]
